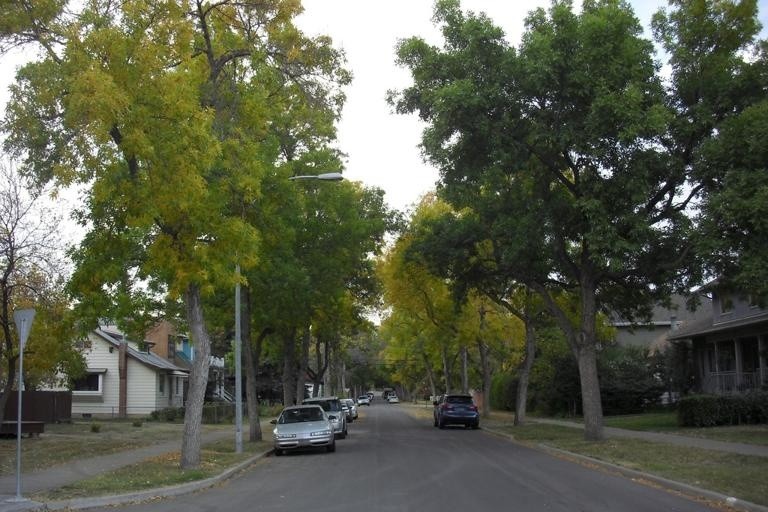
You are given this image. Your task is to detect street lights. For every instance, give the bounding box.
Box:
[234,173,344,455]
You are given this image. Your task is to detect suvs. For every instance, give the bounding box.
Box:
[433,393,480,429]
[301,397,347,439]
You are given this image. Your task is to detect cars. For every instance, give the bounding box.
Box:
[357,393,373,407]
[270,404,335,456]
[383,388,400,403]
[340,398,358,423]
